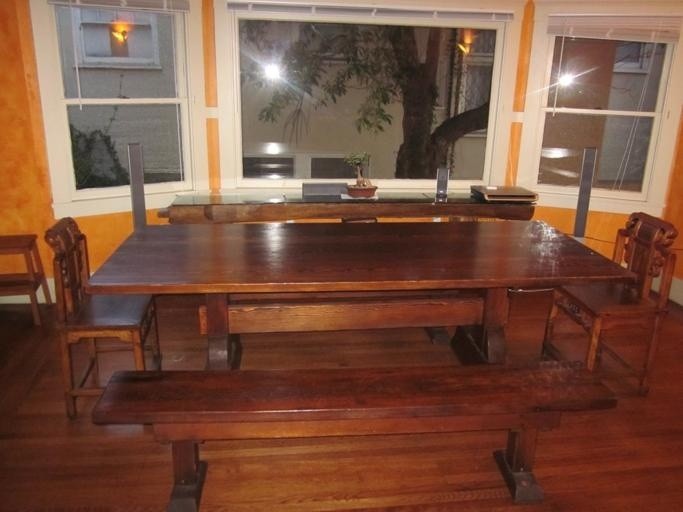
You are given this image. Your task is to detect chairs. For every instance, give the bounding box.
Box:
[537,211,677,396]
[42,215,162,421]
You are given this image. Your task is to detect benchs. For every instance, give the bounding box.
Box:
[90,358,618,512]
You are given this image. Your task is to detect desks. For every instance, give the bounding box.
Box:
[85,189,639,364]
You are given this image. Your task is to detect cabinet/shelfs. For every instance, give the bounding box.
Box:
[0,235,51,326]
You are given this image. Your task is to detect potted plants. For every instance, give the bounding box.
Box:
[346,154,377,196]
